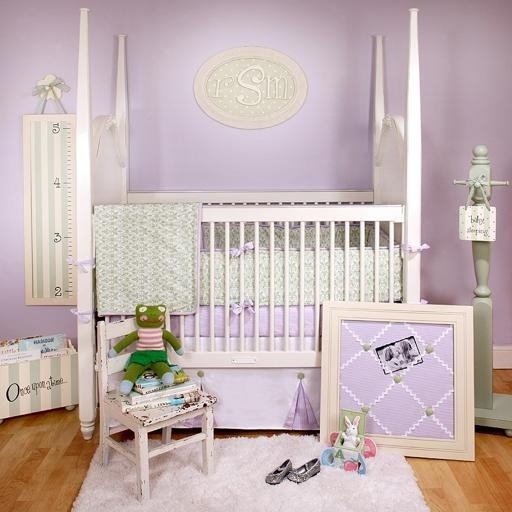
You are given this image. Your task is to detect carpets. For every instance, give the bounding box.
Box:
[70,432,432,512]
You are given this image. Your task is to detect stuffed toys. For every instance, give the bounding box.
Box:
[108,304,185,395]
[340,416,360,448]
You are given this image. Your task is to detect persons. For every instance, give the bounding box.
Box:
[384,338,419,370]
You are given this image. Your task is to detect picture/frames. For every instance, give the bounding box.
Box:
[319,299,476,462]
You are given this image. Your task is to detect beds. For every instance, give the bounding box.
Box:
[73,7,423,442]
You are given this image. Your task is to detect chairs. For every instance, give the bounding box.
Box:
[97,308,218,504]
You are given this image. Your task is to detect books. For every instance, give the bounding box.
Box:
[107,370,199,414]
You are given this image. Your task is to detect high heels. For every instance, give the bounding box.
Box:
[265,458,320,484]
